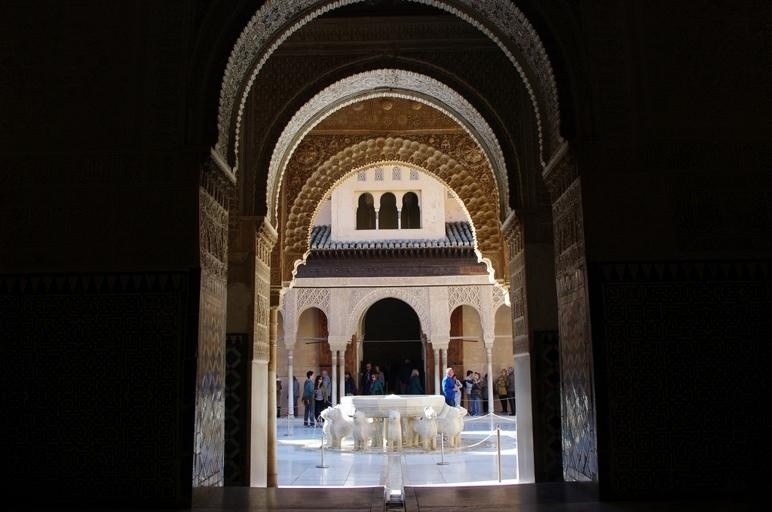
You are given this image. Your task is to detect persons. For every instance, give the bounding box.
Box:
[440,364,515,420]
[276,362,385,428]
[397,357,425,394]
